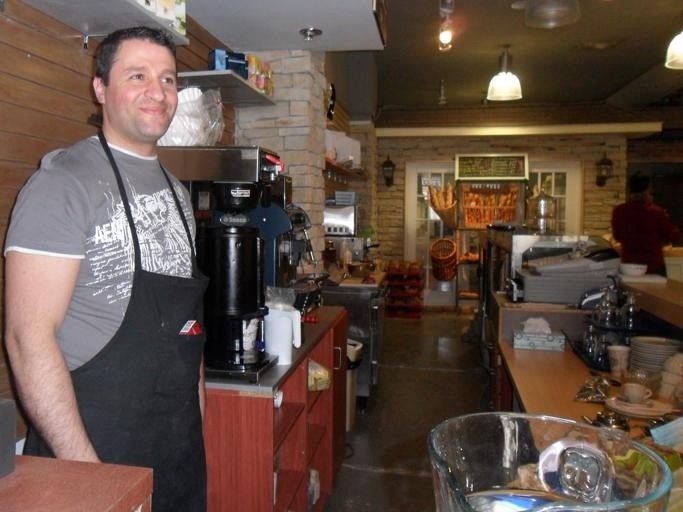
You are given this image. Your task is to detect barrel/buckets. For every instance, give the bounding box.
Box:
[662,246,683,288]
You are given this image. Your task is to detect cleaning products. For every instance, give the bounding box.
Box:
[536,186,548,219]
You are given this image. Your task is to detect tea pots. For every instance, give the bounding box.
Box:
[425,410,673,512]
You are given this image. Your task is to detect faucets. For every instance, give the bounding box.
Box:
[577,289,610,307]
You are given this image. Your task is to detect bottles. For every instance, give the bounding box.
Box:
[246,55,272,97]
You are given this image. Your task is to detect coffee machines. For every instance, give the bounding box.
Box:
[151,144,328,384]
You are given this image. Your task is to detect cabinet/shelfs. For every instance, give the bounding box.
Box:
[0,452,154,511]
[199,305,350,512]
[452,226,484,312]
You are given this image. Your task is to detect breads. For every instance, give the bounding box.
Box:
[464,186,518,225]
[430,181,453,208]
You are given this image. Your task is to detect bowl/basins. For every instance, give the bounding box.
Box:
[347,263,368,278]
[618,263,648,277]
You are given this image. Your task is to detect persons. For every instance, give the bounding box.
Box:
[612,171,683,278]
[2,26,207,512]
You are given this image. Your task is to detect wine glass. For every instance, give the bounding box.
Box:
[580,286,640,365]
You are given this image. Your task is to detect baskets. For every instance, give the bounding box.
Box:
[430,192,458,229]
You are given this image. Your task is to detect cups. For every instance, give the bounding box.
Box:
[622,382,651,403]
[326,170,353,186]
[606,344,629,374]
[261,301,302,367]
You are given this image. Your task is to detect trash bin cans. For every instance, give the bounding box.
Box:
[346,338,365,432]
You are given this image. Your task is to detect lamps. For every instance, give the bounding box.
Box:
[592,151,614,186]
[484,45,524,101]
[523,1,580,30]
[664,31,683,71]
[380,152,396,188]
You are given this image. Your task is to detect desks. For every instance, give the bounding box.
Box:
[487,289,620,413]
[493,339,683,512]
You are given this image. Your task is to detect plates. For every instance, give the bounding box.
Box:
[628,336,681,375]
[601,397,677,420]
